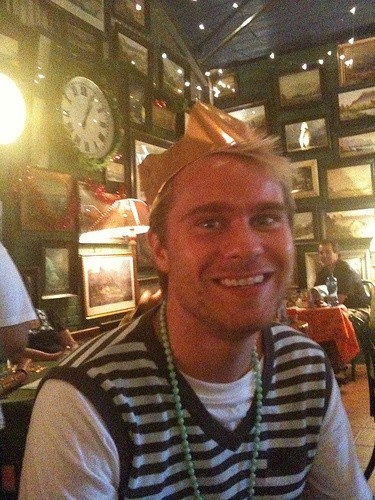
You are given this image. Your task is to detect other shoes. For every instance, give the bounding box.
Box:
[335,367,352,379]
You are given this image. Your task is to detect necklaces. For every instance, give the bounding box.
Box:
[160,300,263,500]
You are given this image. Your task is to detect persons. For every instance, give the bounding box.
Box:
[21,272,80,361]
[314,239,369,381]
[18,101,371,500]
[0,242,40,430]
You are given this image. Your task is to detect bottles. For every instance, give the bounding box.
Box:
[326,273,339,307]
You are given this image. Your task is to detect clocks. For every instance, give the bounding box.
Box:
[49,64,124,170]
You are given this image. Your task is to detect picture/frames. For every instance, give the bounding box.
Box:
[276,34,375,290]
[0,0,274,321]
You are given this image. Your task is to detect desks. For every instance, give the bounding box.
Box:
[287,305,360,381]
[0,359,61,497]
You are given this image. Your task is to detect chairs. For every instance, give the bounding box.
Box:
[346,312,375,479]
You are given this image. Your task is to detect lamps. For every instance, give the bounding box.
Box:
[85,197,152,305]
